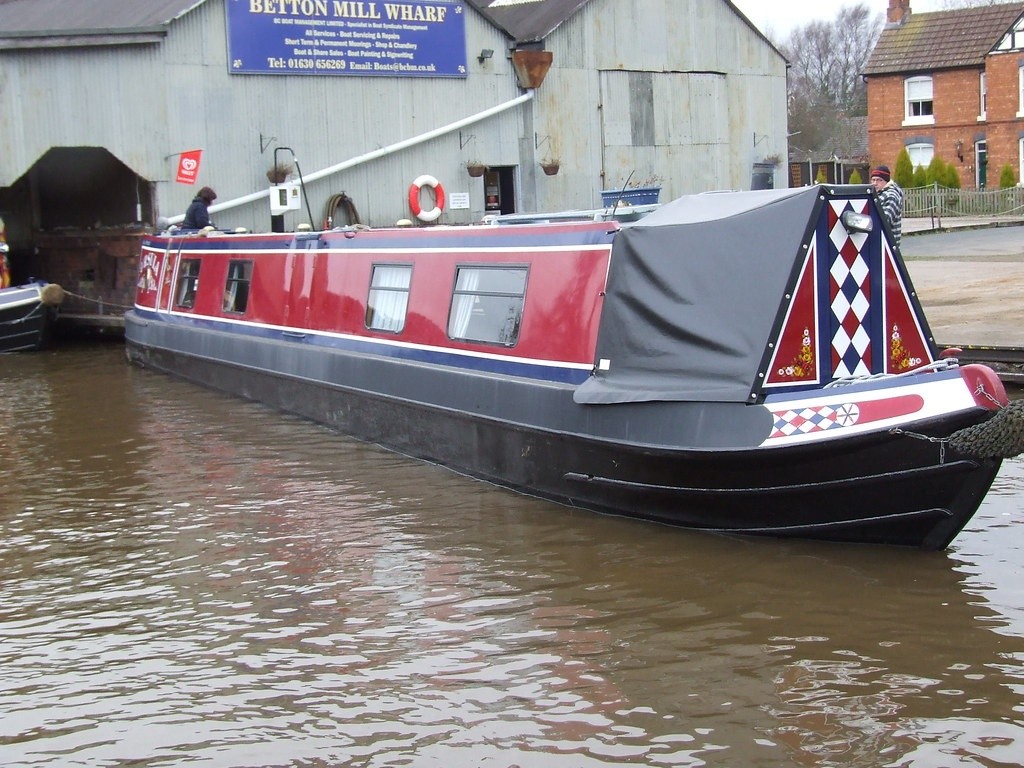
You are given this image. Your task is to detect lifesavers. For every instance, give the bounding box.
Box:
[408,174,445,222]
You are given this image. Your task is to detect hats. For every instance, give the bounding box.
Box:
[871,165,890,182]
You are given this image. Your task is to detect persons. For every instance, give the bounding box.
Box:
[872,166,904,251]
[181,187,217,304]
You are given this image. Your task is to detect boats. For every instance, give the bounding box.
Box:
[0,277,66,355]
[126,180,1024,556]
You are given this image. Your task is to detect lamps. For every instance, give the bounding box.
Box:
[478,48,493,63]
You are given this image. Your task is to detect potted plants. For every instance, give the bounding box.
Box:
[460,160,488,178]
[266,160,293,183]
[536,156,561,175]
